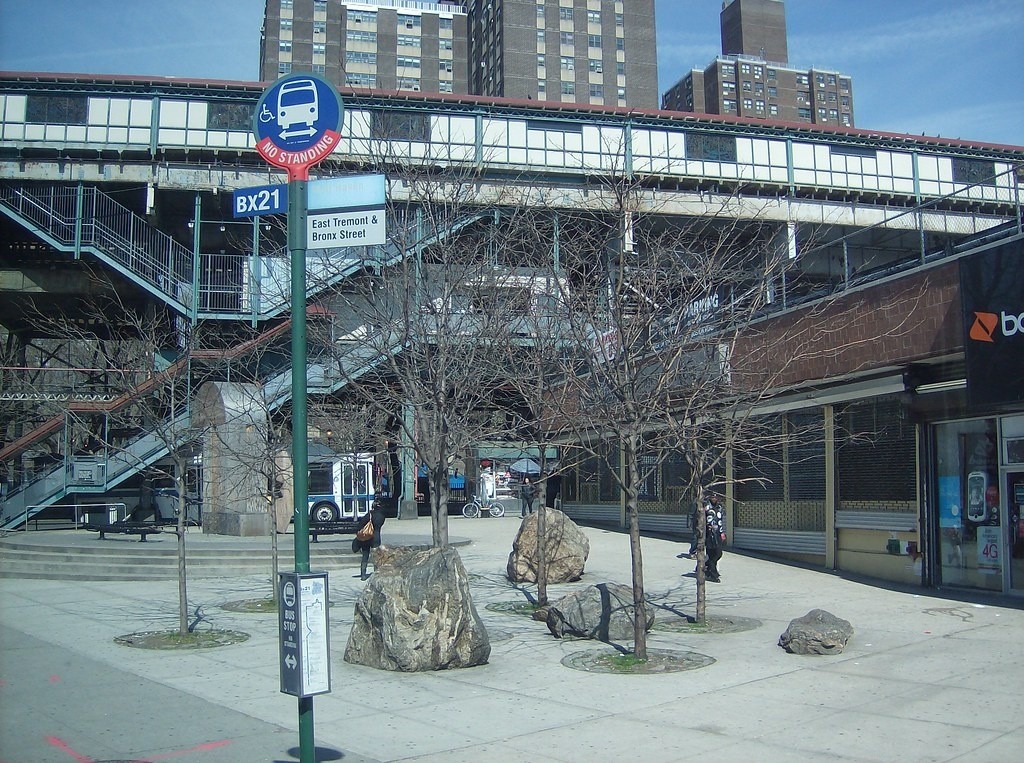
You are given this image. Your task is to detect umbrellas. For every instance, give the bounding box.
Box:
[510,458,542,475]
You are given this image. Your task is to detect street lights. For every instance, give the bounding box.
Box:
[327,428,332,448]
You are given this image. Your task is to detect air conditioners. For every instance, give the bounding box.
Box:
[355,15,362,22]
[489,76,492,81]
[260,26,264,32]
[596,68,602,73]
[406,19,413,25]
[314,28,320,33]
[261,35,265,39]
[481,62,485,67]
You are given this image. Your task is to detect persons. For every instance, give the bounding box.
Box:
[518,476,536,519]
[352,500,387,582]
[687,492,705,561]
[694,491,726,583]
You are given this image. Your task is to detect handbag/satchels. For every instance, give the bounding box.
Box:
[357,513,374,541]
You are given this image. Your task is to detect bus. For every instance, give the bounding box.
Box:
[72,444,383,528]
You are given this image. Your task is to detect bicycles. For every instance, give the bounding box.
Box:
[462,494,504,518]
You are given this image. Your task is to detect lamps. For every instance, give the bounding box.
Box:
[220,224,226,231]
[188,222,195,228]
[264,224,272,230]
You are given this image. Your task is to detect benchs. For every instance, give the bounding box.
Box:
[86,524,162,543]
[308,521,358,543]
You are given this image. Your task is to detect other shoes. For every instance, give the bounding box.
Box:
[362,574,370,581]
[707,568,717,577]
[689,553,697,560]
[519,516,524,519]
